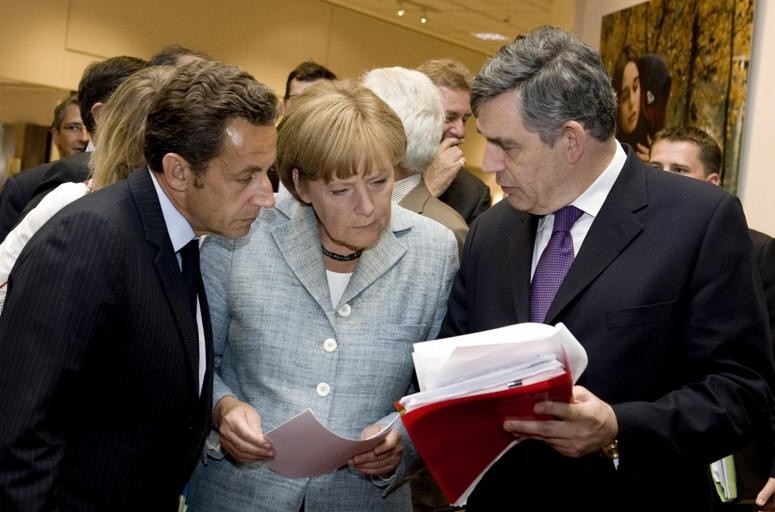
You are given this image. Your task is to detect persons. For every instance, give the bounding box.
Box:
[649,126,775,507]
[1,63,281,511]
[410,27,775,511]
[268,63,337,196]
[609,44,672,164]
[359,58,493,245]
[0,43,218,315]
[183,80,460,510]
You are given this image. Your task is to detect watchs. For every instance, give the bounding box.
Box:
[604,443,619,459]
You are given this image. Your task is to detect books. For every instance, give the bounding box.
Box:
[395,361,574,506]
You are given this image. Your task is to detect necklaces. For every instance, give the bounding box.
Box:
[322,244,365,261]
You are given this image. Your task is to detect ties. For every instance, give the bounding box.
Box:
[180,239,200,318]
[529,206,584,323]
